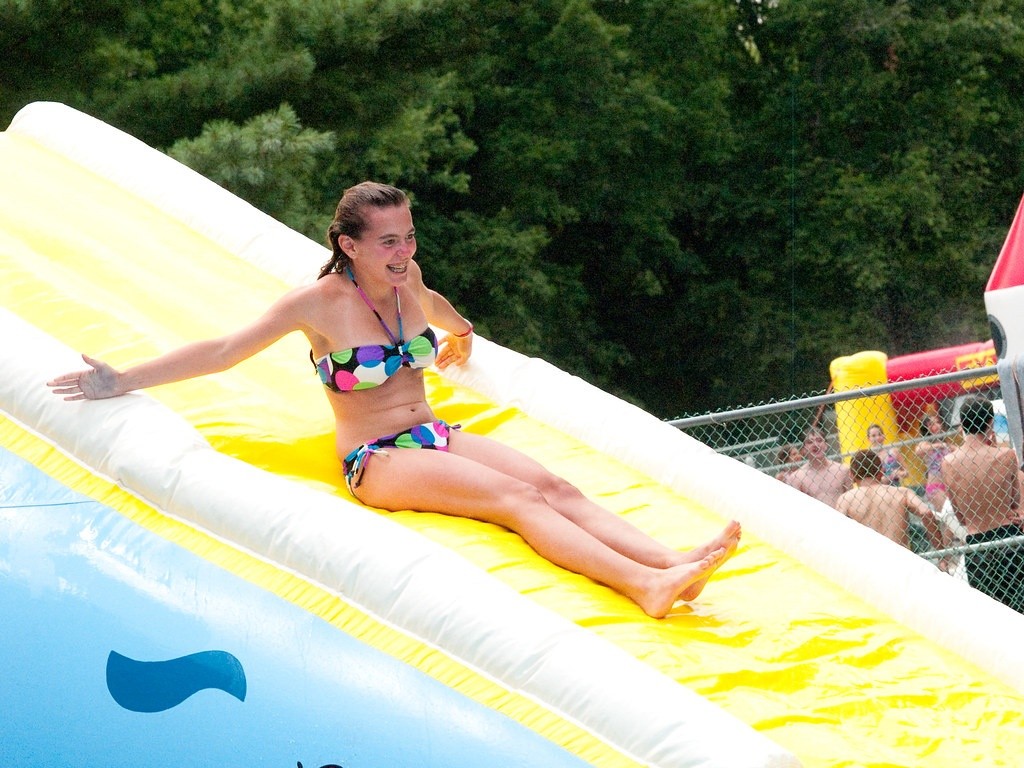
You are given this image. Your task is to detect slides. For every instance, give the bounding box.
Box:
[0,91,1024,768]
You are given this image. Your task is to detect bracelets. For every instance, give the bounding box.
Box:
[453,320,473,337]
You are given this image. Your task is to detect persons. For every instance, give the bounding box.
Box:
[46,180,741,619]
[776,397,1024,613]
